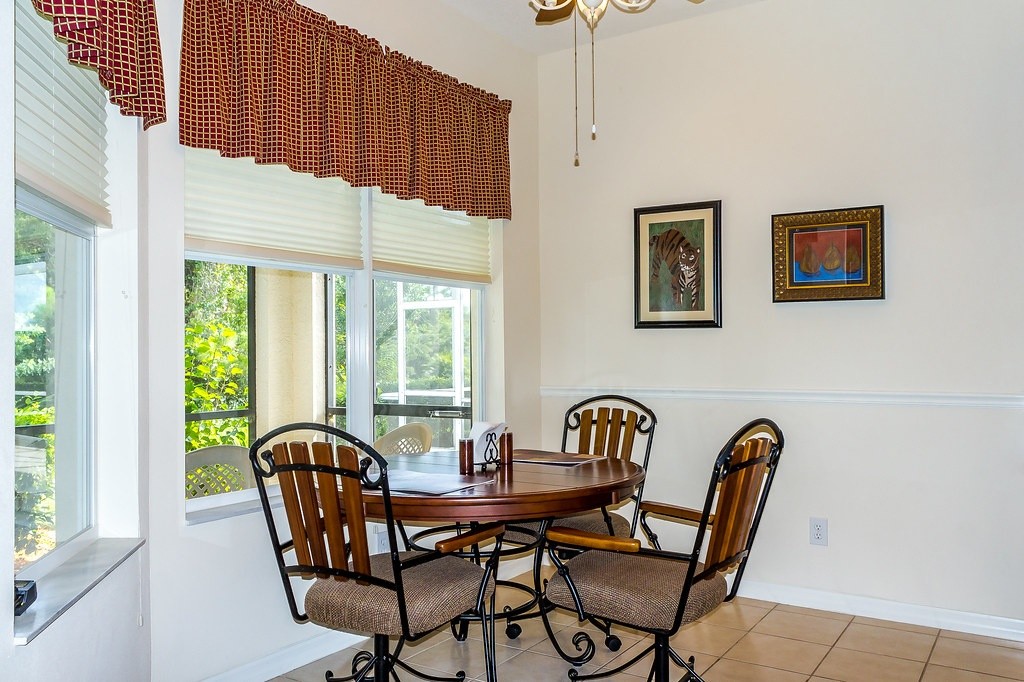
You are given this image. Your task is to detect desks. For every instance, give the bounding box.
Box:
[312,448,646,682]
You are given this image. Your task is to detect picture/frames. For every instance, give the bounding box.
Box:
[632,199,722,329]
[772,204,886,302]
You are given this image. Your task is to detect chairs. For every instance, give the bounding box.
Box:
[361,422,433,457]
[500,394,658,651]
[544,417,785,682]
[248,422,497,682]
[185,444,270,500]
